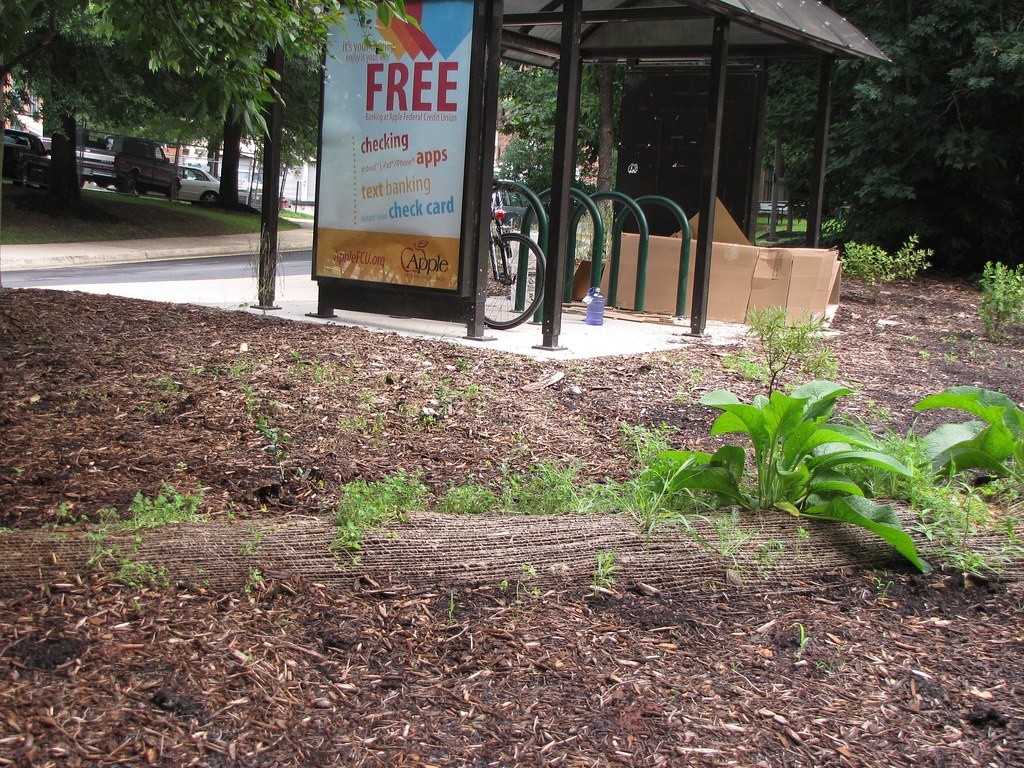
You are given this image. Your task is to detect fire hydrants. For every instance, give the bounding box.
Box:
[280,197,287,208]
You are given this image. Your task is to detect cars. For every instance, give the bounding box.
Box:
[2,129,54,185]
[173,164,220,203]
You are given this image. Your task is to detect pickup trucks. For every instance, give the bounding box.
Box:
[76,128,185,199]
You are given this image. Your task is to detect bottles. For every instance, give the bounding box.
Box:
[586,287,604,325]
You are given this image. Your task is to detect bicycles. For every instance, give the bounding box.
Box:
[481,175,545,330]
